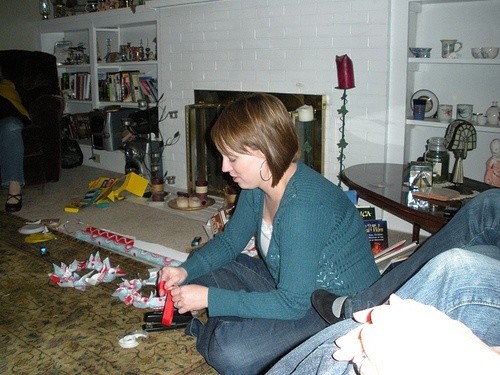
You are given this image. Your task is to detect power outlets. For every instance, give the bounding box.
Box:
[92,154,100,162]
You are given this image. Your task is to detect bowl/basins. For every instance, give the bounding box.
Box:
[408,48,432,58]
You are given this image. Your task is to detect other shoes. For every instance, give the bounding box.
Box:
[5,189,23,212]
[311,289,349,324]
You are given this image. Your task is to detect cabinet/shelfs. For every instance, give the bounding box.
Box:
[37,6,160,174]
[406,58,500,133]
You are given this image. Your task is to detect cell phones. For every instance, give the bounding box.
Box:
[40,246,47,255]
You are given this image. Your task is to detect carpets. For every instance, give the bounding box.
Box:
[0,210,225,375]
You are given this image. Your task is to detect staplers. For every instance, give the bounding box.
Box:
[142,311,193,332]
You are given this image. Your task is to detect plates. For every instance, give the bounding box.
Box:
[410,89,440,118]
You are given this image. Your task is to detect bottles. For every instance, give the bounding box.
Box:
[486,102,500,124]
[424,137,450,184]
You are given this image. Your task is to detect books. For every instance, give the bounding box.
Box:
[61,70,158,103]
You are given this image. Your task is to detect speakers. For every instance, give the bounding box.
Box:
[88,111,130,152]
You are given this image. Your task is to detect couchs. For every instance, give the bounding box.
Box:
[0,50,63,184]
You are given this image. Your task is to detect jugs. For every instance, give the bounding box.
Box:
[440,40,462,58]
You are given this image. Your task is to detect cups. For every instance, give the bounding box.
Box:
[151,177,163,201]
[471,48,482,58]
[481,47,499,59]
[227,188,237,208]
[412,99,427,121]
[195,181,208,201]
[437,104,453,123]
[456,104,473,122]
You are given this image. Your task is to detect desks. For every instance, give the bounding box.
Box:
[339,163,500,245]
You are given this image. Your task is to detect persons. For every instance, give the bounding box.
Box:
[484,139,500,187]
[156,93,382,375]
[259,189,500,375]
[0,73,31,212]
